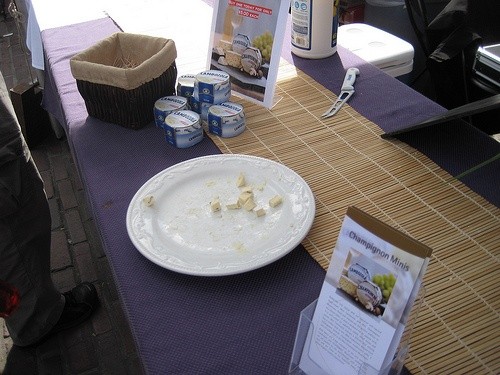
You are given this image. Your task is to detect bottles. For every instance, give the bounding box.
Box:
[290,0,340,60]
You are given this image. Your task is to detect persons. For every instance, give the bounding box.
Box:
[0,70,97,348]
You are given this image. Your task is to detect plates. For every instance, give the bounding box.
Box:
[126,154,316,277]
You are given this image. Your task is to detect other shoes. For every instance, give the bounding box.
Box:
[13,281,99,351]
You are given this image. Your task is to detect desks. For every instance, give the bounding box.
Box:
[26,0,500,375]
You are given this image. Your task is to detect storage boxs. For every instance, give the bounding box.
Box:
[337,23,415,87]
[472,42,500,89]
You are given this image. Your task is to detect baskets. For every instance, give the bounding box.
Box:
[70,32,179,130]
